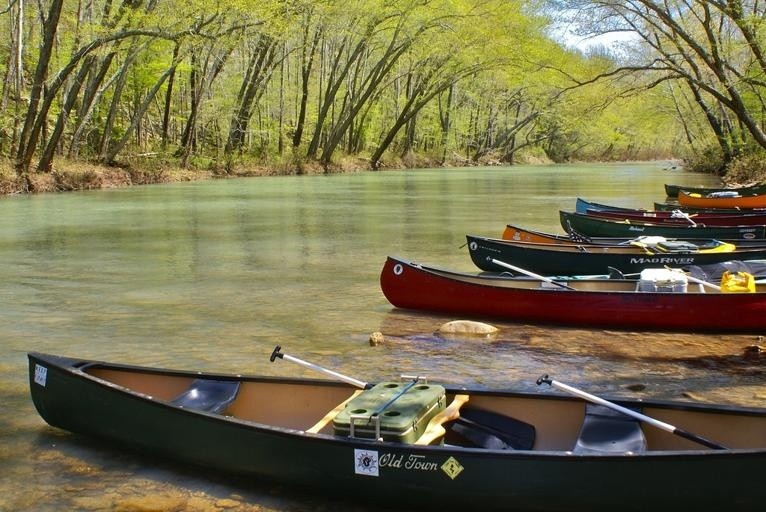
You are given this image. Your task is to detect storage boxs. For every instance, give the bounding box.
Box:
[639,268,688,292]
[656,241,698,253]
[332,382,447,446]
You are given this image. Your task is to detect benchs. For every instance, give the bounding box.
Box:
[542,281,567,289]
[167,378,241,414]
[572,403,647,453]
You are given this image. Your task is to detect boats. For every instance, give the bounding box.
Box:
[25,348,765,502]
